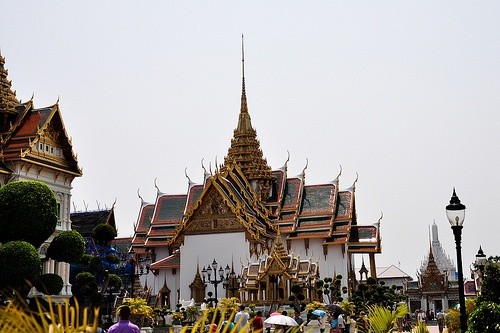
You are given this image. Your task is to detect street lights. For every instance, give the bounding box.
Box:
[222,264,243,297]
[201,258,231,306]
[445,186,469,333]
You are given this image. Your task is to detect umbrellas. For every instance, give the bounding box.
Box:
[312,310,326,316]
[324,305,345,314]
[264,315,299,326]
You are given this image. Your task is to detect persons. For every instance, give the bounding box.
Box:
[318,313,368,333]
[107,305,140,333]
[201,299,206,309]
[207,305,305,333]
[436,308,444,333]
[415,308,448,328]
